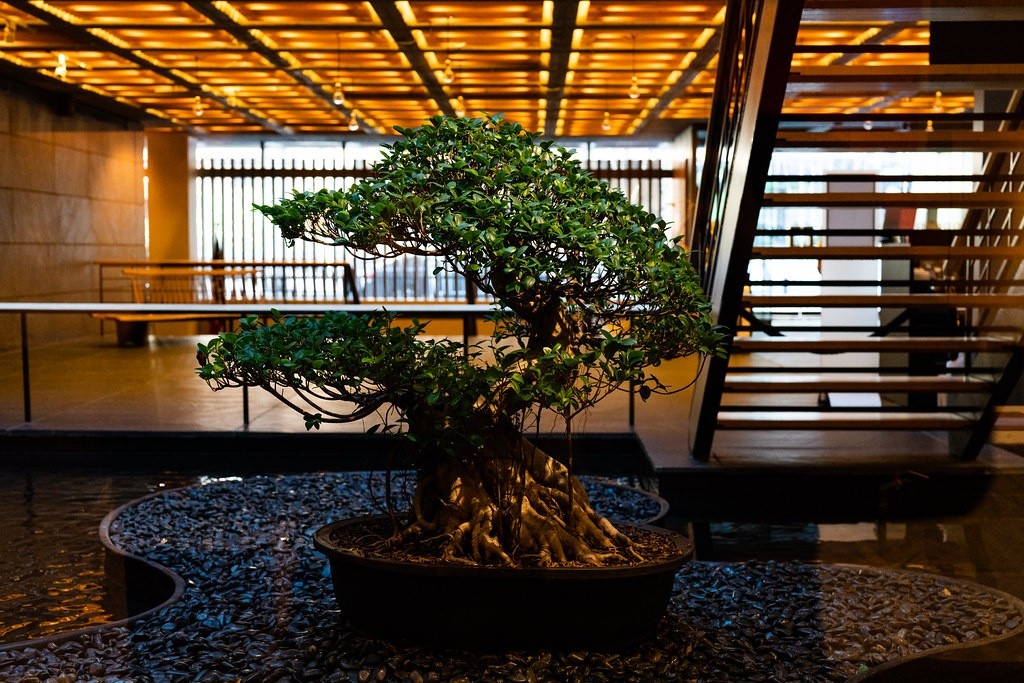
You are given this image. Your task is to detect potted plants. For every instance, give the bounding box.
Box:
[192,117,729,652]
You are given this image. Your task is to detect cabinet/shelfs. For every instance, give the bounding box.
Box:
[877,232,972,410]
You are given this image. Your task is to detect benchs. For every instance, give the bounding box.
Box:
[91,267,264,347]
